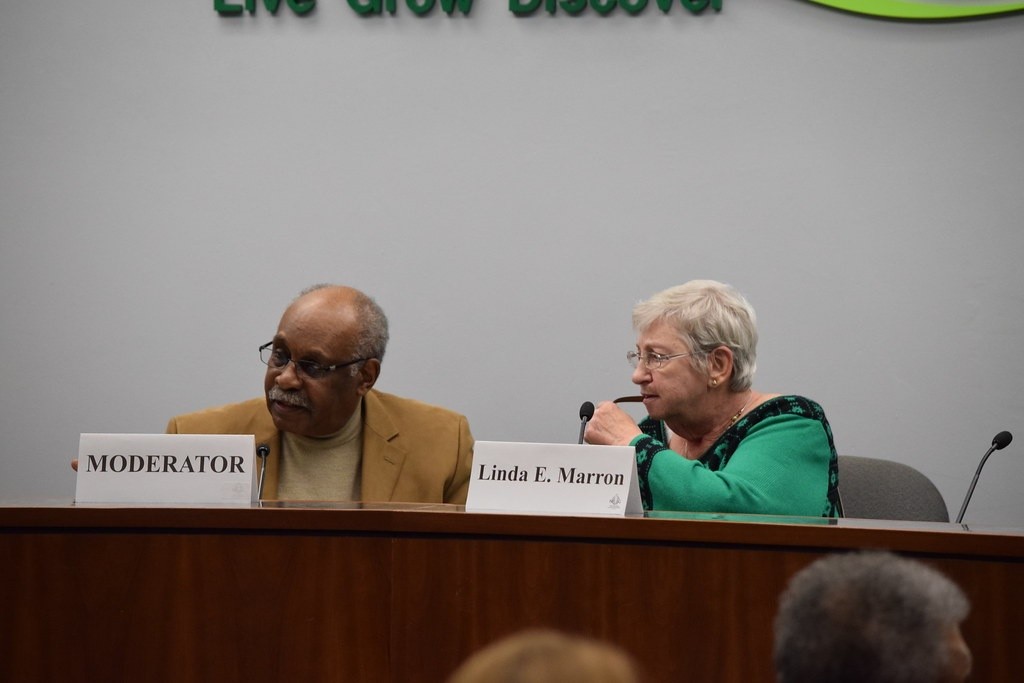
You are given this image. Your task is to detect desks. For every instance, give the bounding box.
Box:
[0,500,1024,683]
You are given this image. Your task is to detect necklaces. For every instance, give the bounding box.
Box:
[683,393,753,460]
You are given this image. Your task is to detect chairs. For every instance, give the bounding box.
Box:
[838,455,951,522]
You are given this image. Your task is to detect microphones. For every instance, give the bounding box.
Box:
[256,443,271,500]
[954,431,1013,524]
[576,400,596,443]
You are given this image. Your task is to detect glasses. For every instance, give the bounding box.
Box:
[259,341,374,379]
[627,350,713,370]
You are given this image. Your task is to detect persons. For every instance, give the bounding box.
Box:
[772,554,977,683]
[582,279,846,518]
[71,282,474,502]
[446,628,640,682]
